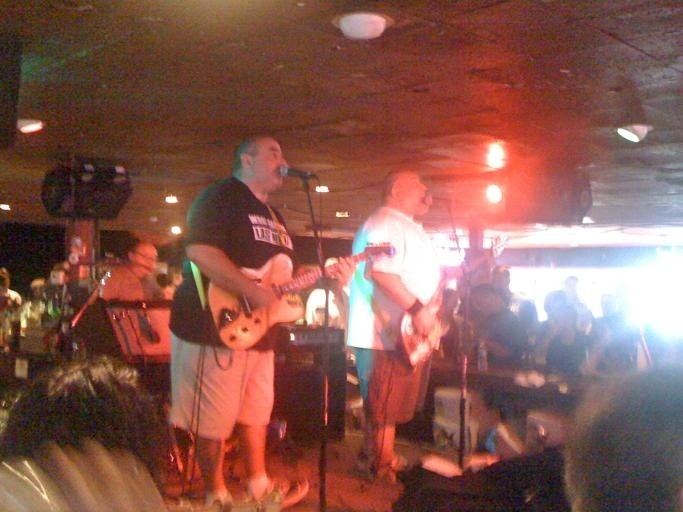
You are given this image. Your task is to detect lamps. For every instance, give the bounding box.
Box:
[330,12,396,40]
[16,117,44,134]
[617,125,656,143]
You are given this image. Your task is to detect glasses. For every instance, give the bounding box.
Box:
[135,251,155,263]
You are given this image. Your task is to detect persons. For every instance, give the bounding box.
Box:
[165,131,356,512]
[345,166,496,488]
[1,238,681,510]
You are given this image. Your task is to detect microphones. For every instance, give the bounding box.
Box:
[278,166,311,178]
[424,196,456,205]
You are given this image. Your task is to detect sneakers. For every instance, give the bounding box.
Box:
[206,477,315,512]
[350,450,408,478]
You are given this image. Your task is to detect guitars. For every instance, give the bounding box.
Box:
[400,237,507,368]
[207,243,396,351]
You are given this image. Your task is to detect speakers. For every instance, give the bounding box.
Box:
[506,169,593,226]
[41,169,133,220]
[270,324,344,443]
